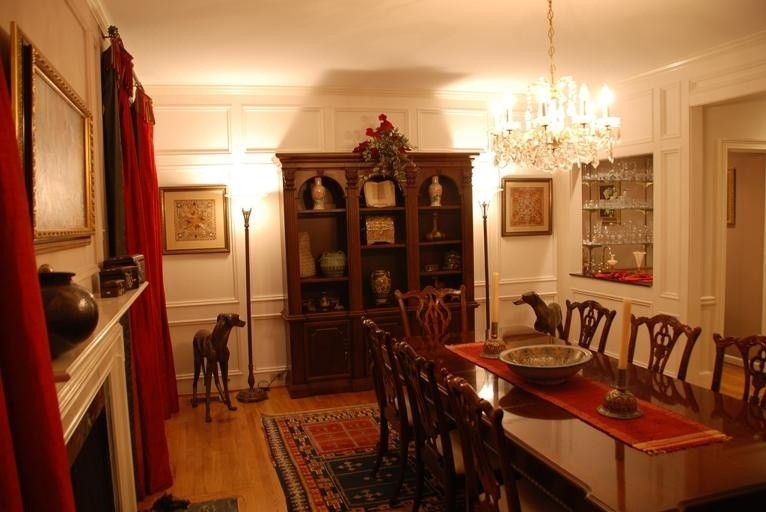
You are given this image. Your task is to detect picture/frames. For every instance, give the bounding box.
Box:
[11,21,96,255]
[501,178,553,236]
[727,167,735,225]
[160,185,230,255]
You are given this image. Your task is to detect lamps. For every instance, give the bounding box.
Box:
[472,163,500,329]
[229,163,279,403]
[490,0,623,174]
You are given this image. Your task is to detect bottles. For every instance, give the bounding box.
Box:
[426,212,446,242]
[319,250,345,276]
[429,177,442,206]
[371,268,391,303]
[312,177,326,209]
[37,271,98,361]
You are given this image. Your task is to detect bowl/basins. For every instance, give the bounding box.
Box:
[499,344,594,386]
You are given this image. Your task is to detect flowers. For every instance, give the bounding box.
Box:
[353,114,416,190]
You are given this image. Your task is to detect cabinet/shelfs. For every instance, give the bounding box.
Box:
[582,180,653,272]
[275,152,481,399]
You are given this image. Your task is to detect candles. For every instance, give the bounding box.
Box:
[492,272,498,322]
[618,298,631,368]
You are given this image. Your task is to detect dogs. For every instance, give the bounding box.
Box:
[192,313,246,422]
[512,290,564,338]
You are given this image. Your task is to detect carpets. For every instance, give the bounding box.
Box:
[261,403,497,512]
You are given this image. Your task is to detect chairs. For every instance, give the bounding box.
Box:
[394,284,469,337]
[358,316,523,512]
[564,300,766,408]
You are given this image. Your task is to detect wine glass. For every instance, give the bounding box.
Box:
[584,221,651,244]
